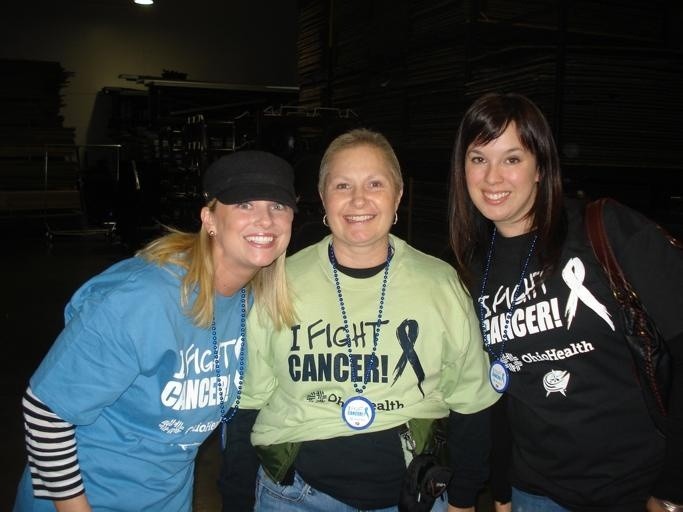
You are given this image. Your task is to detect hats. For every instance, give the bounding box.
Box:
[202,150,297,210]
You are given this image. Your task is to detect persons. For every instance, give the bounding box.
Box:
[9,147,299,512]
[236,127,508,512]
[429,90,683,512]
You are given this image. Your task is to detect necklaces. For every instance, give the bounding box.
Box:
[323,237,403,434]
[474,226,545,400]
[185,271,256,452]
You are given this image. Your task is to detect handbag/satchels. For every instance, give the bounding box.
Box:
[399,453,454,512]
[585,196,682,440]
